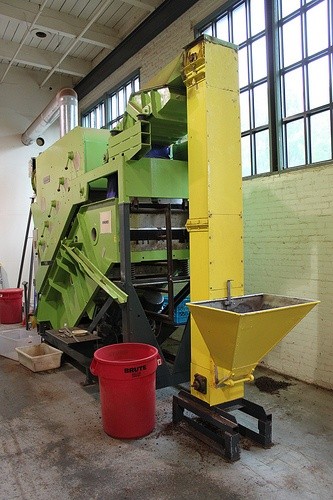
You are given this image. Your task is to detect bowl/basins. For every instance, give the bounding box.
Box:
[72,330,88,337]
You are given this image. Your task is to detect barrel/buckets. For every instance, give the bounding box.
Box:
[0,288,23,324]
[89,343,162,438]
